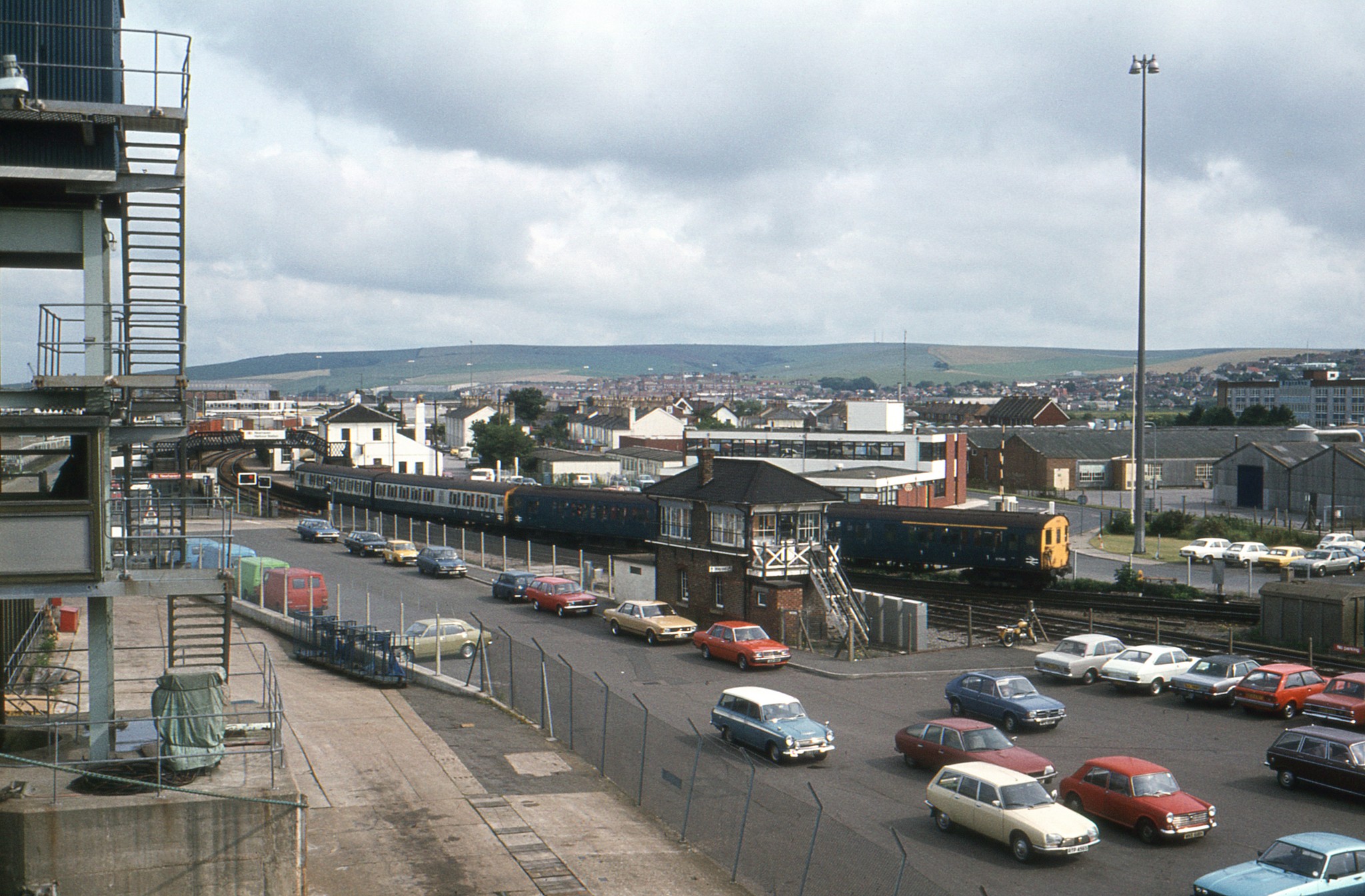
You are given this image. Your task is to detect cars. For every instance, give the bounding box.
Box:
[944,669,1067,732]
[1168,655,1278,708]
[1262,724,1365,801]
[1033,633,1151,684]
[1192,831,1365,896]
[923,761,1101,862]
[1059,756,1217,845]
[709,686,836,766]
[1301,671,1365,732]
[1179,532,1365,578]
[297,517,792,671]
[1097,644,1209,696]
[391,618,492,662]
[894,717,1058,783]
[1233,664,1350,719]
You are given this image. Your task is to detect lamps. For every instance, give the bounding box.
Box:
[0,53,30,98]
[108,230,117,251]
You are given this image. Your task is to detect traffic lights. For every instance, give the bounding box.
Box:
[240,474,255,484]
[260,477,269,487]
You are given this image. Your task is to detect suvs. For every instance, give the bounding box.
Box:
[170,536,330,615]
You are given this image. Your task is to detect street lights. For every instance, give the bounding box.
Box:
[1130,52,1159,555]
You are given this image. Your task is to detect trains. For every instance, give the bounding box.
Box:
[294,461,1072,590]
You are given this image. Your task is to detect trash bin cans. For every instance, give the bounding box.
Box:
[60,606,79,633]
[1280,567,1295,582]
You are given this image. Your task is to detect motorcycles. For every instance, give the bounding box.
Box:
[996,608,1038,647]
